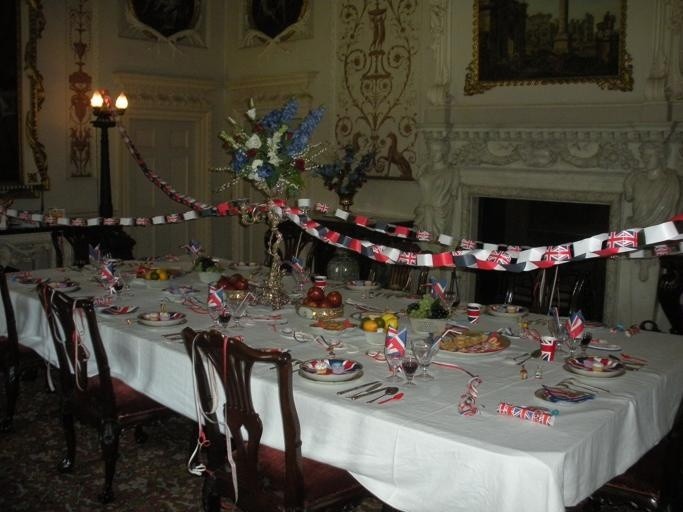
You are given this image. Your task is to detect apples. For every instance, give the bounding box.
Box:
[382,314,400,330]
[304,286,342,308]
[217,274,248,290]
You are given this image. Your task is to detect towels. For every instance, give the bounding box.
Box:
[98,262,115,281]
[429,273,447,301]
[565,308,585,341]
[86,241,102,262]
[206,283,227,311]
[381,324,408,360]
[100,301,140,318]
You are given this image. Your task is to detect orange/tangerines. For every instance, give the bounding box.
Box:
[374,317,384,328]
[151,273,158,280]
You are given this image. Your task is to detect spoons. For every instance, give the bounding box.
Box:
[516,348,541,367]
[366,386,402,404]
[378,393,405,406]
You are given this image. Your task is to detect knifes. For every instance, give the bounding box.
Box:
[346,381,383,400]
[351,386,390,400]
[336,380,380,396]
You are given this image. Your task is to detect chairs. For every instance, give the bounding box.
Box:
[35,281,175,502]
[501,257,595,318]
[179,328,396,512]
[375,243,436,299]
[0,266,53,432]
[50,224,123,269]
[580,390,681,512]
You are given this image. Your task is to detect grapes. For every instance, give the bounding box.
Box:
[407,293,449,320]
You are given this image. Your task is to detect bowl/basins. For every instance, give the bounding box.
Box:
[294,298,345,320]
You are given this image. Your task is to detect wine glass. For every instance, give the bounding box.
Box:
[292,266,311,294]
[444,290,457,318]
[410,338,437,381]
[383,346,404,384]
[215,305,232,336]
[226,295,248,329]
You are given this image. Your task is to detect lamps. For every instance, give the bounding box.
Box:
[86,86,135,260]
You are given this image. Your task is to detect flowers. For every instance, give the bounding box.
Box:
[314,141,375,199]
[206,90,326,196]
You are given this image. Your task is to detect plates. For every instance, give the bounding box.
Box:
[484,304,530,319]
[587,342,622,352]
[44,281,79,292]
[437,334,511,357]
[533,387,596,404]
[345,280,381,291]
[298,358,365,384]
[11,276,42,289]
[135,310,188,328]
[159,286,200,296]
[94,305,140,317]
[229,261,262,272]
[563,355,626,379]
[350,311,400,322]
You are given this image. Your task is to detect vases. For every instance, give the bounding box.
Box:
[337,199,354,216]
[252,179,297,308]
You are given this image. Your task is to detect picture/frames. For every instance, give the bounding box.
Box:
[117,0,208,53]
[236,0,315,57]
[461,0,635,99]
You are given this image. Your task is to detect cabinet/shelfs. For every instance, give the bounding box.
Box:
[262,210,415,290]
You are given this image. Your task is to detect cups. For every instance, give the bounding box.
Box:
[547,319,567,341]
[314,275,327,291]
[539,336,559,362]
[467,302,481,327]
